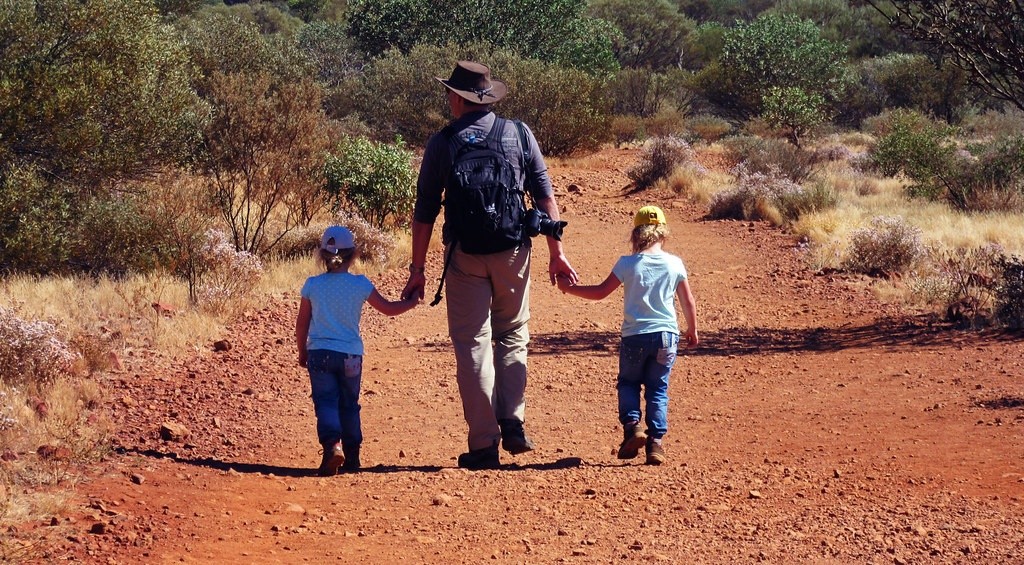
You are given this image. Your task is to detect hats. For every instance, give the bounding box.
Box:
[435,60,507,105]
[634,206,667,226]
[321,226,355,254]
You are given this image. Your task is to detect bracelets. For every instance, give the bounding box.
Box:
[409,263,424,274]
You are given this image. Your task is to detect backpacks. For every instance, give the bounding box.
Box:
[439,115,534,255]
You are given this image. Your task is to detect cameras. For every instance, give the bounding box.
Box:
[524,207,568,240]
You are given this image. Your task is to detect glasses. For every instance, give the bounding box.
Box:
[445,86,451,94]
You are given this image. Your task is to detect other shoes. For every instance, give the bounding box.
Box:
[457,444,501,469]
[618,422,647,459]
[645,442,664,463]
[318,442,346,476]
[344,460,361,472]
[501,424,535,455]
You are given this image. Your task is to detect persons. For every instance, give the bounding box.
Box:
[556,206,699,462]
[296,226,419,477]
[401,63,578,471]
